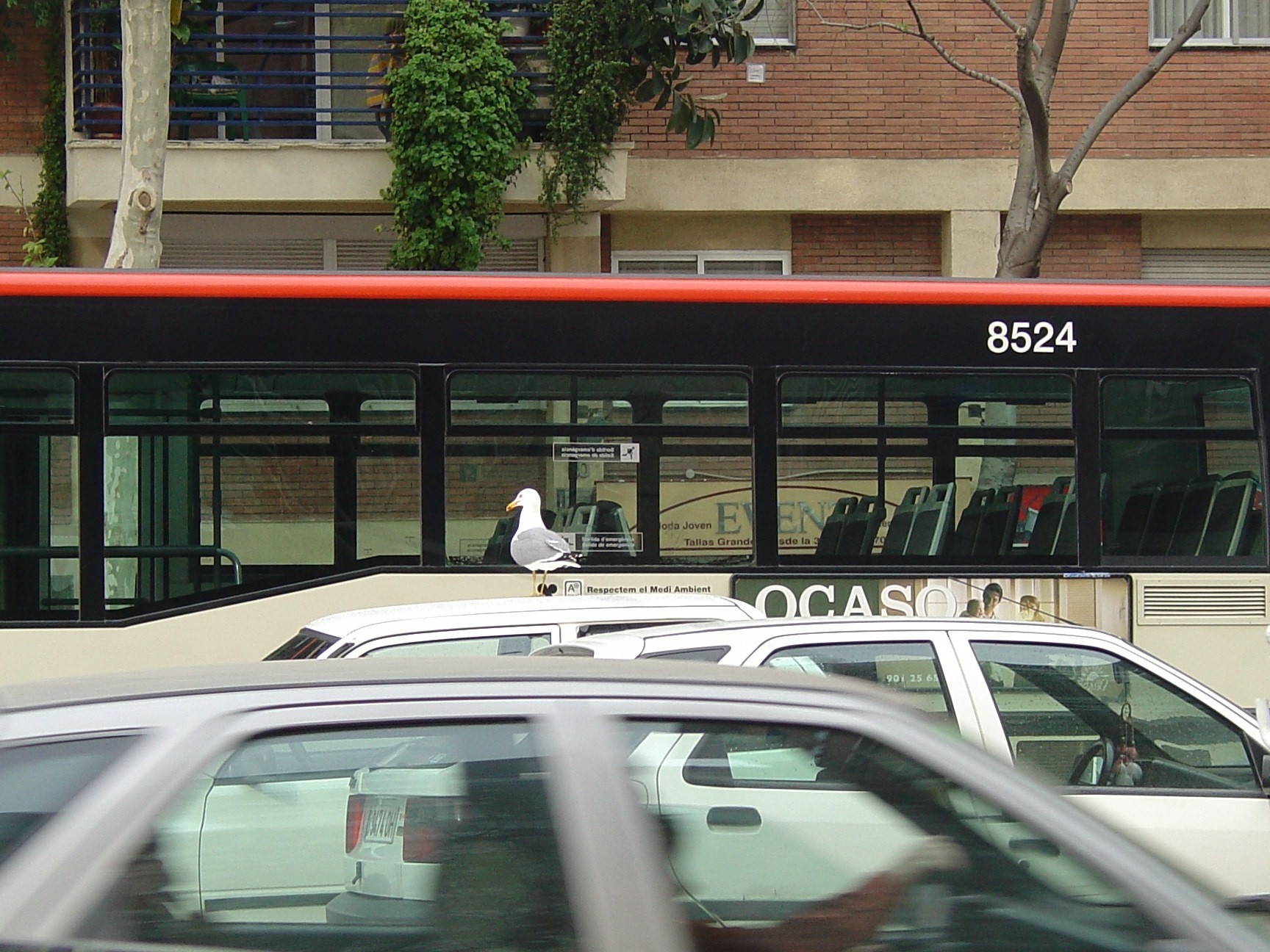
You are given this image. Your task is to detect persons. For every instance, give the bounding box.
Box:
[1019,595,1046,621]
[639,812,969,952]
[958,583,1002,619]
[383,4,411,45]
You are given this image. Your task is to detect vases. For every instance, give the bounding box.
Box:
[87,102,122,140]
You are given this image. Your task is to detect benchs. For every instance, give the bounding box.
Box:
[815,471,1259,557]
[484,500,637,560]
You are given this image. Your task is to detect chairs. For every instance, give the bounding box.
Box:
[424,830,579,952]
[175,60,249,142]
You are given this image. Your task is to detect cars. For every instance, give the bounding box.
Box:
[0,640,1270,952]
[317,616,1270,942]
[118,582,857,922]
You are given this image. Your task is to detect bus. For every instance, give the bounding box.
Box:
[0,265,1270,776]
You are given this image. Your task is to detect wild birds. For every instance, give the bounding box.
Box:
[506,487,580,596]
